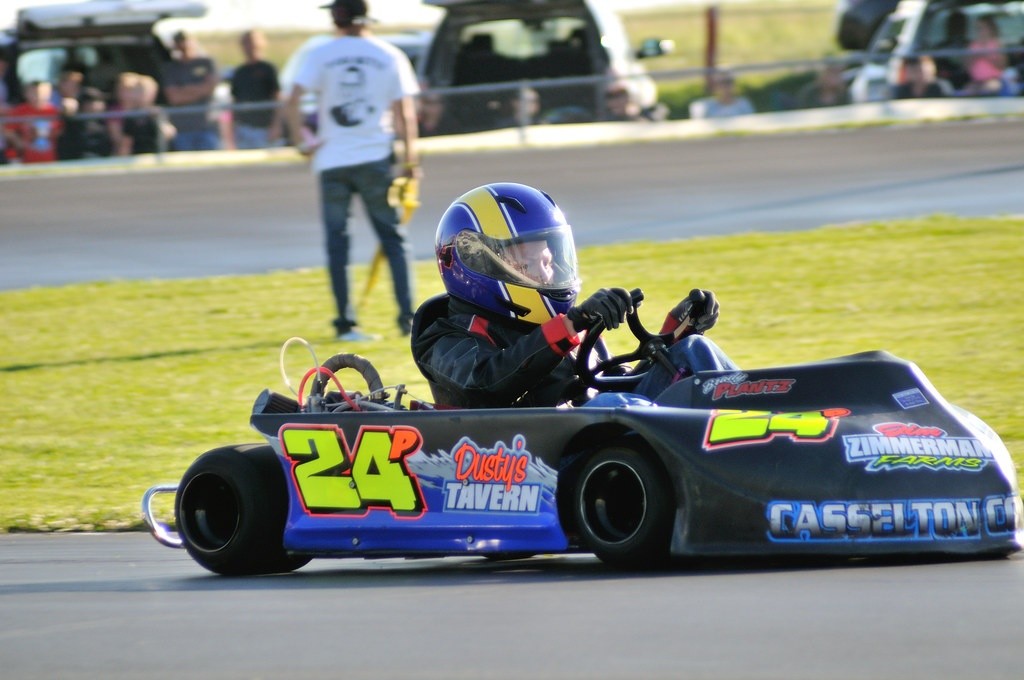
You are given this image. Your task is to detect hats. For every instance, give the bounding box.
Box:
[320,0,366,22]
[75,87,111,101]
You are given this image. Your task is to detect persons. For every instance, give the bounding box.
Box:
[281,0,419,340]
[412,182,740,412]
[1,15,1020,165]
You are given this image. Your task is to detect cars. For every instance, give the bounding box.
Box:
[414,0,673,136]
[1,0,208,132]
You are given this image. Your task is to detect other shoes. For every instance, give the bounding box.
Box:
[336,324,352,341]
[400,321,412,336]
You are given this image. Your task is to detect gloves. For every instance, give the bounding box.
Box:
[659,290,719,348]
[568,288,633,333]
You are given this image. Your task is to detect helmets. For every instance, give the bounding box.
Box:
[435,182,581,326]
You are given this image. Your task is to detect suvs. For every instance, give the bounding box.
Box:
[848,1,1023,103]
